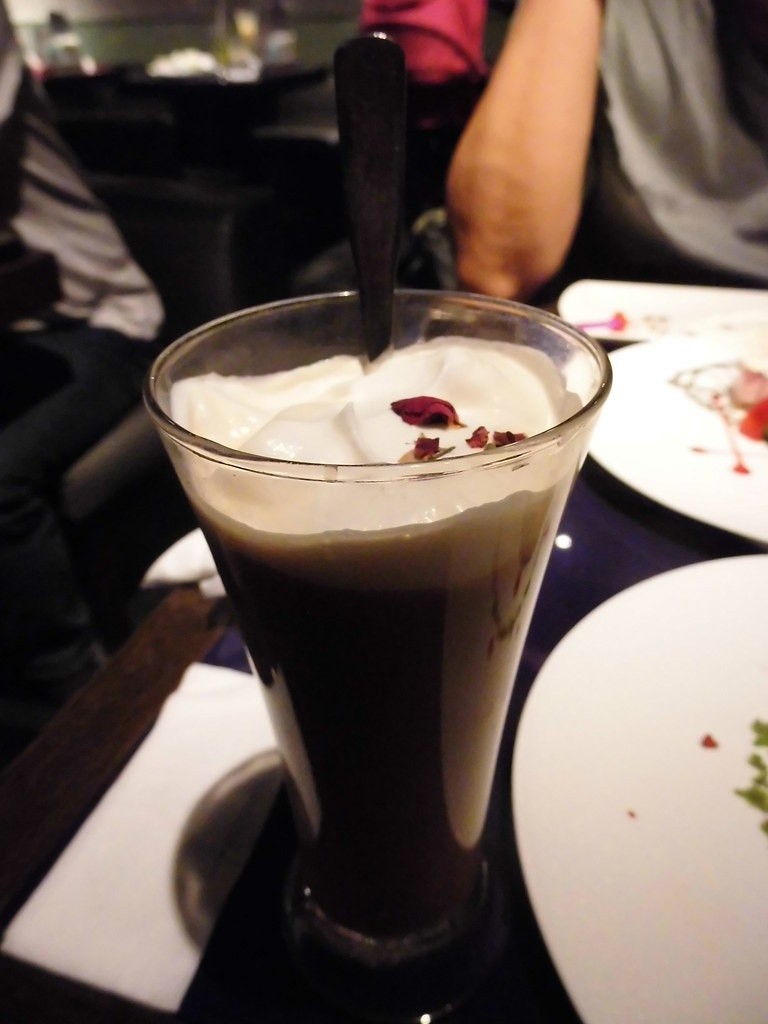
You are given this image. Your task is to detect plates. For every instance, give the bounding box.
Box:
[513,553,768,1024]
[558,279,768,342]
[578,335,768,542]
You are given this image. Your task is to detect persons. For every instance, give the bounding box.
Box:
[357,0,488,132]
[445,0,768,304]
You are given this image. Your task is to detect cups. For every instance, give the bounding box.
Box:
[144,286,611,1023]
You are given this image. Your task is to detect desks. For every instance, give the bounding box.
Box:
[1,298,768,1024]
[41,60,328,176]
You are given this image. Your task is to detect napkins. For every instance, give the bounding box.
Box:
[2,660,285,1014]
